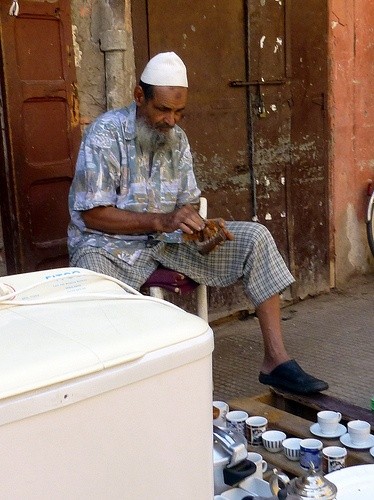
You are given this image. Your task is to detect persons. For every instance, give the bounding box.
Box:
[68,52,329,393]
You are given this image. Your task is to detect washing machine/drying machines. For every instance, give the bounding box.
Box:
[0,266,215,500]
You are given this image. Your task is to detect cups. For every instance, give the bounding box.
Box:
[282,438,302,461]
[247,452,267,480]
[300,438,323,471]
[225,410,249,436]
[213,401,229,428]
[322,446,347,476]
[317,411,342,435]
[261,430,286,453]
[245,416,268,448]
[347,420,370,446]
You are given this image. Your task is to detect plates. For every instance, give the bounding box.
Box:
[369,447,374,457]
[309,423,347,438]
[323,464,374,500]
[340,433,374,449]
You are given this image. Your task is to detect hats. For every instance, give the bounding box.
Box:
[140,52,188,88]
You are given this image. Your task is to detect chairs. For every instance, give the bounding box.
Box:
[142,197,208,324]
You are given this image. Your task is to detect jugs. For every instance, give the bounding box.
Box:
[269,460,337,500]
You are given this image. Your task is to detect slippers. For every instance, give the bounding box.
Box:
[260,358,327,392]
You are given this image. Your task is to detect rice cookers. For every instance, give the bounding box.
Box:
[213,423,257,495]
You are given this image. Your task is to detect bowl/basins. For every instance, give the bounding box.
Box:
[220,487,255,500]
[240,477,281,500]
